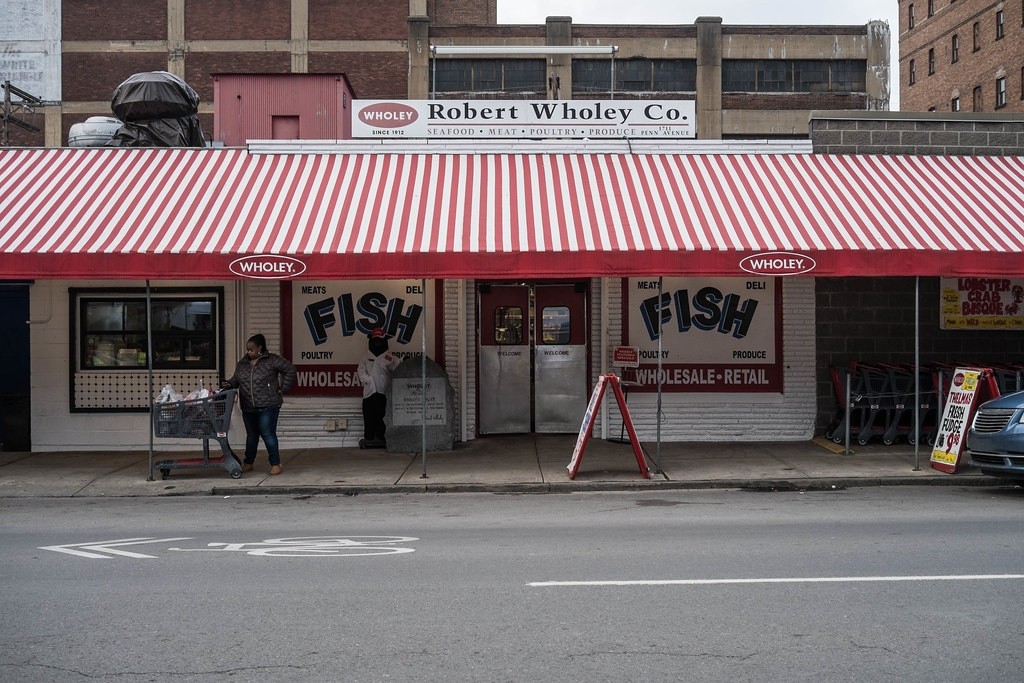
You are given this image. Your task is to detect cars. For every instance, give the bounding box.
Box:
[966,390,1024,487]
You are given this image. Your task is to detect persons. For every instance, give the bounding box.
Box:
[224,333,298,475]
[357,328,409,451]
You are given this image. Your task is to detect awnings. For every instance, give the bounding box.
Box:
[0,147,1024,283]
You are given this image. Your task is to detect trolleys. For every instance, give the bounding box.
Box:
[152,389,244,479]
[826,360,1024,446]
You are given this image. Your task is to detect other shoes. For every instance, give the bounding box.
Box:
[270,464,282,474]
[242,463,253,472]
